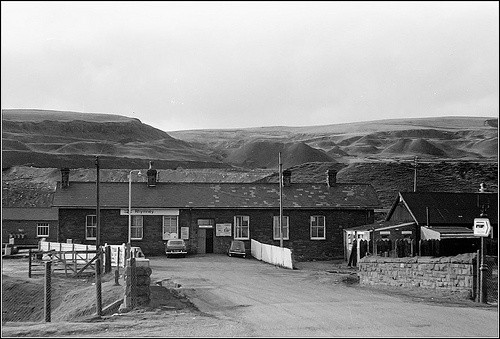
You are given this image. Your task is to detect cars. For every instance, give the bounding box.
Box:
[226,241,246,259]
[165,239,187,257]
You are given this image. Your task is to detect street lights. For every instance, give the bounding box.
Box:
[128,170,141,245]
